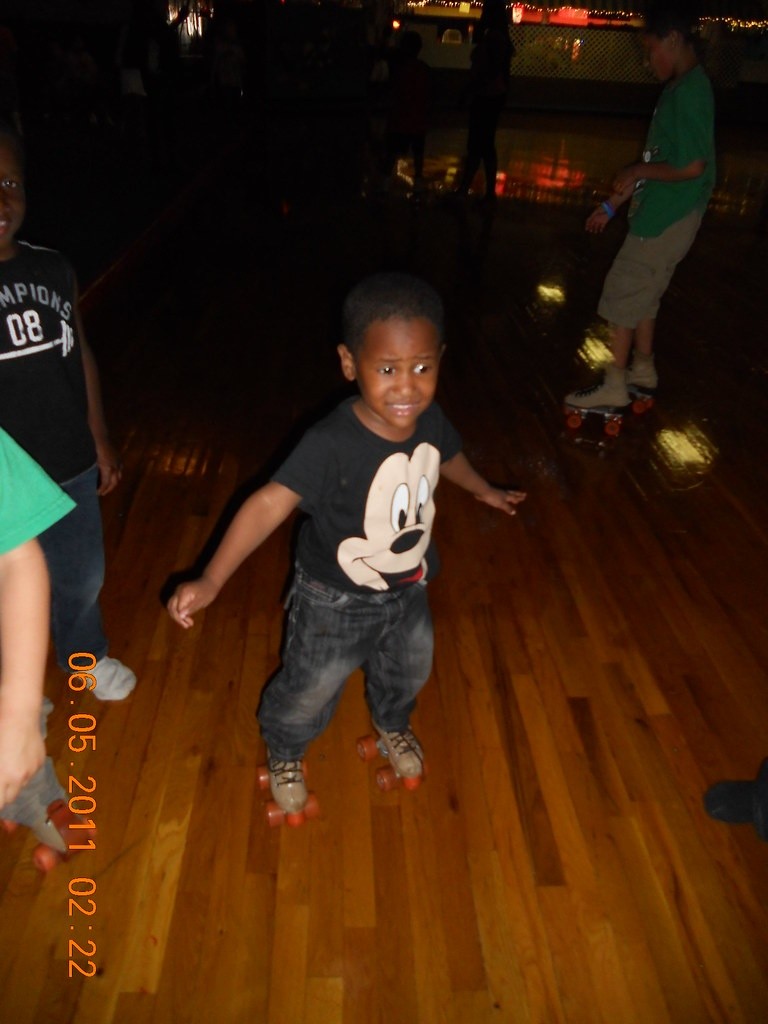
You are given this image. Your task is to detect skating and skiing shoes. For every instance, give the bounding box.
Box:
[627,351,657,413]
[258,747,319,827]
[565,363,632,437]
[0,756,99,873]
[357,716,428,792]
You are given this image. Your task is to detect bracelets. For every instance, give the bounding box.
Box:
[600,200,614,218]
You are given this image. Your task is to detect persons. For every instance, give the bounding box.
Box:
[168,273,527,817]
[441,0,514,203]
[566,8,716,408]
[2,139,137,832]
[375,30,431,205]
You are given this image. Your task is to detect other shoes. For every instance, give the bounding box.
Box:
[704,780,754,824]
